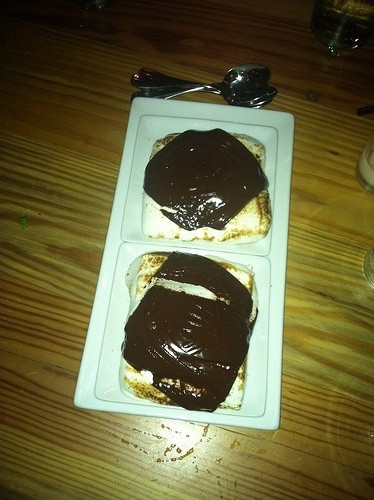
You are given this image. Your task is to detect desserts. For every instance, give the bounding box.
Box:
[143,129,273,244]
[121,251,258,413]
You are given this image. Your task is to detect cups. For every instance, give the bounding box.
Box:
[310,0,374,50]
[355,135,374,193]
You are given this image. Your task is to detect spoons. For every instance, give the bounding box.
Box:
[131,63,277,109]
